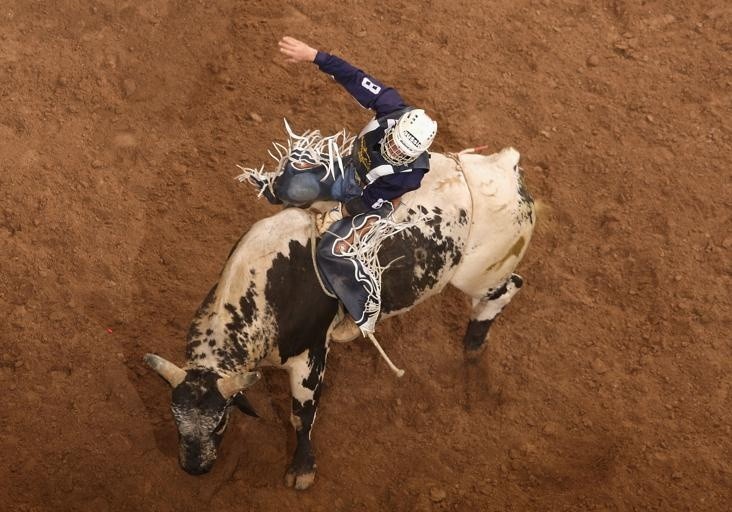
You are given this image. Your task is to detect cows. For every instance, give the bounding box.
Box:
[142,147,550,492]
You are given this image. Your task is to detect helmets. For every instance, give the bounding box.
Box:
[390,107,439,163]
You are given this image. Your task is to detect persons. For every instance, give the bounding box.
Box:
[233,34,440,344]
